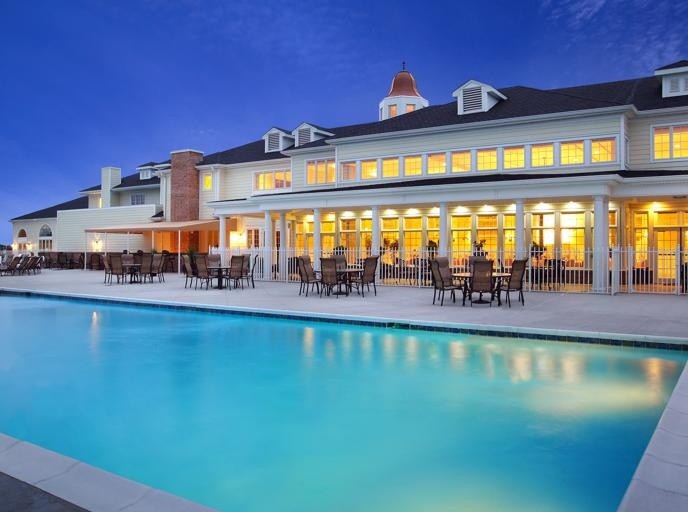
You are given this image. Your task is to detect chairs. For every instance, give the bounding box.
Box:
[182,252,259,291]
[0,254,41,275]
[288,256,381,299]
[39,251,175,273]
[99,252,167,284]
[382,256,434,280]
[426,255,529,308]
